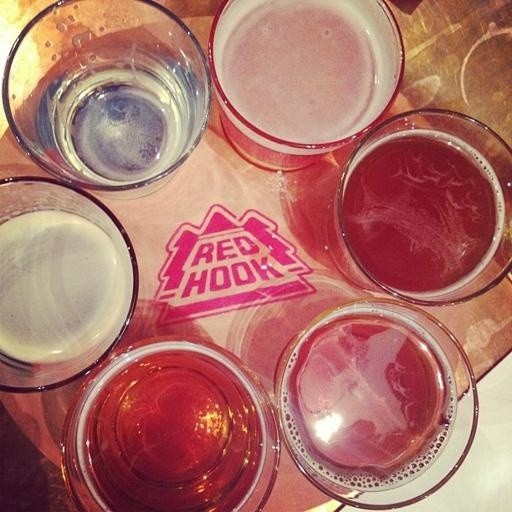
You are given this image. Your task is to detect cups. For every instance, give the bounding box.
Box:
[1,0,211,201]
[62,333,283,511]
[325,109,511,307]
[0,177,139,394]
[208,0,404,172]
[274,298,480,512]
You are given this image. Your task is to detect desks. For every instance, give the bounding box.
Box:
[0,0,512,512]
